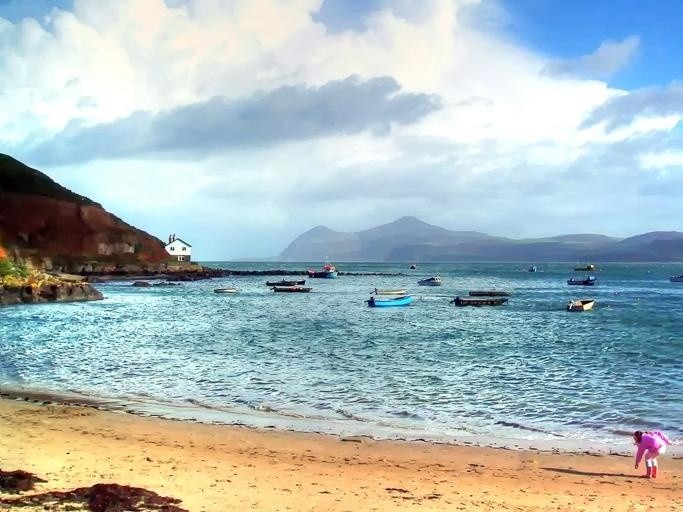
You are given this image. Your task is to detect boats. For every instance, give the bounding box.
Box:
[371,288,410,295]
[364,295,414,307]
[566,299,594,311]
[409,263,416,269]
[417,274,441,286]
[527,265,537,272]
[307,263,338,279]
[567,274,596,286]
[270,285,312,294]
[450,295,509,308]
[669,274,682,282]
[468,290,513,297]
[574,263,596,272]
[213,287,236,295]
[265,277,305,286]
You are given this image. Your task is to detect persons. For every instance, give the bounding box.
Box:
[632,430,674,478]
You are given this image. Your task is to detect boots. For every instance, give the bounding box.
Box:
[652,466,656,478]
[643,467,652,479]
